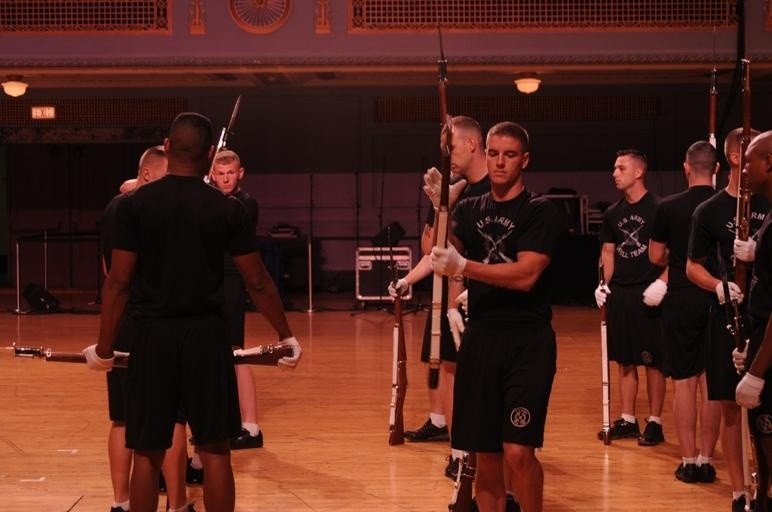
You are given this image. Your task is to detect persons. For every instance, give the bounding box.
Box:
[731,129,771,511]
[647,139,724,485]
[403,168,462,444]
[419,118,560,512]
[387,112,523,511]
[684,126,771,511]
[594,148,668,447]
[159,443,205,493]
[79,109,305,511]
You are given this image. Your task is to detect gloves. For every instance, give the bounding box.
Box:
[446,288,468,352]
[594,280,612,309]
[422,167,468,214]
[388,278,409,303]
[716,236,766,409]
[277,336,302,371]
[642,278,669,307]
[430,239,467,283]
[83,344,115,372]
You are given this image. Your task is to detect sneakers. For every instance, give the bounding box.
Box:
[444,452,522,511]
[110,456,204,512]
[598,418,772,512]
[404,417,449,441]
[231,426,263,448]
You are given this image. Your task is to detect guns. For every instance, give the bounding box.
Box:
[708,66,718,193]
[428,6,451,389]
[598,258,611,446]
[734,60,751,318]
[715,246,761,512]
[202,96,240,186]
[1,343,294,368]
[387,226,407,446]
[448,450,477,511]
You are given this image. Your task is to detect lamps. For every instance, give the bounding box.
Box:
[513,71,543,95]
[1,74,30,98]
